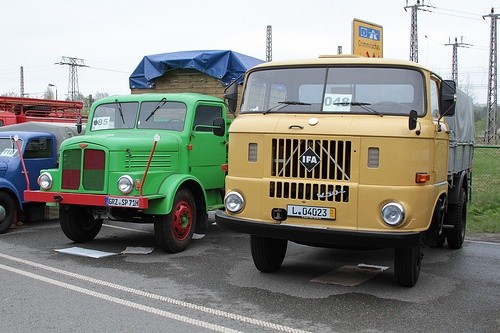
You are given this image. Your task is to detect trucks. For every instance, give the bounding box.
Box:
[0,95,87,233]
[13,92,233,254]
[214,52,476,288]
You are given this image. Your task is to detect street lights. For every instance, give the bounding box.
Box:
[49,83,58,101]
